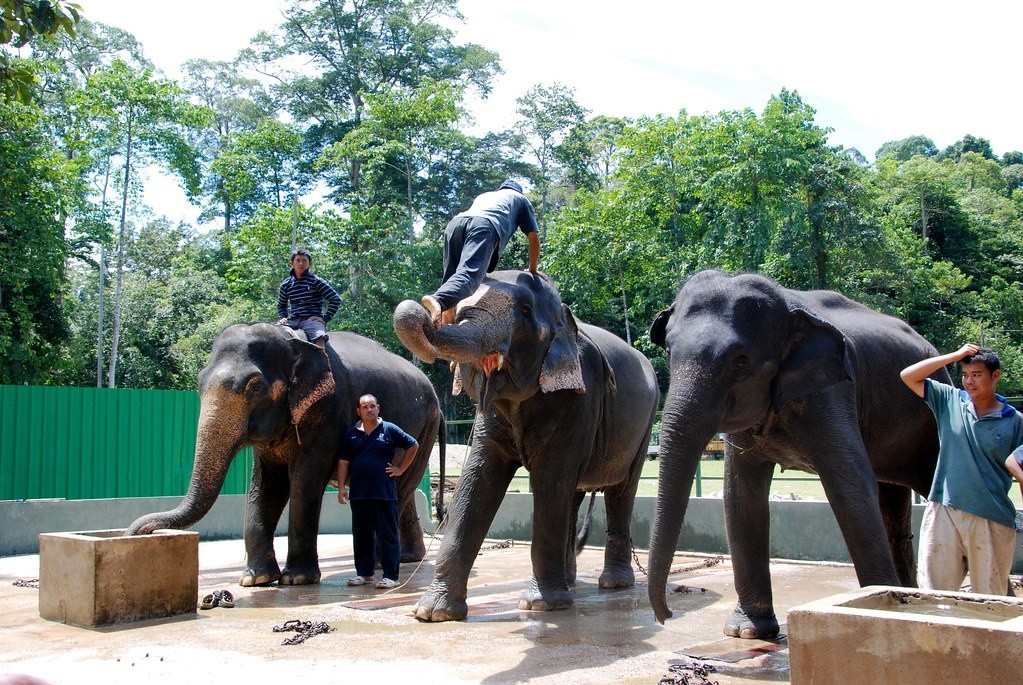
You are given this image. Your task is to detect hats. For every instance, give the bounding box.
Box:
[499,180,523,194]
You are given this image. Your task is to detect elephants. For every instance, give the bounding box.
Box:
[121,321,447,586]
[392,268,661,624]
[650,269,957,640]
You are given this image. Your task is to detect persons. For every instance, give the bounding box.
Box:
[338,394,419,588]
[423,179,541,328]
[277,249,342,352]
[900,343,1023,596]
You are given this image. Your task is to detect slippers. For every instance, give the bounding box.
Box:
[376,578,400,588]
[346,576,374,585]
[201,590,234,609]
